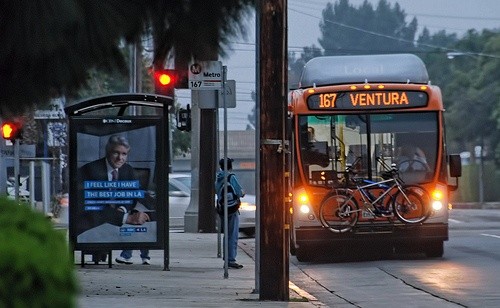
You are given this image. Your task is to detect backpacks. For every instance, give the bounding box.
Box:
[216,173,241,215]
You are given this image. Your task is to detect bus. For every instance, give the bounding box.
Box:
[287,54,464,264]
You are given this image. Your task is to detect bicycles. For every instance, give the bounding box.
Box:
[319,157,433,234]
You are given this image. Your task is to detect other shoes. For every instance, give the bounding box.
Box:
[116,257,133,264]
[228,262,243,269]
[143,259,150,264]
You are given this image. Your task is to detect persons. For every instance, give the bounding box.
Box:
[115,248,151,265]
[80,133,150,224]
[216,158,246,268]
[398,143,430,170]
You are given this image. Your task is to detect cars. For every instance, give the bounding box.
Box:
[53,170,257,237]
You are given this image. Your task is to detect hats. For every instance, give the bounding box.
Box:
[219,157,234,164]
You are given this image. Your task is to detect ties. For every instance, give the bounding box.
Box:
[111,170,118,209]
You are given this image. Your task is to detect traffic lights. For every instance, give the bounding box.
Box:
[154,70,177,97]
[1,121,20,141]
